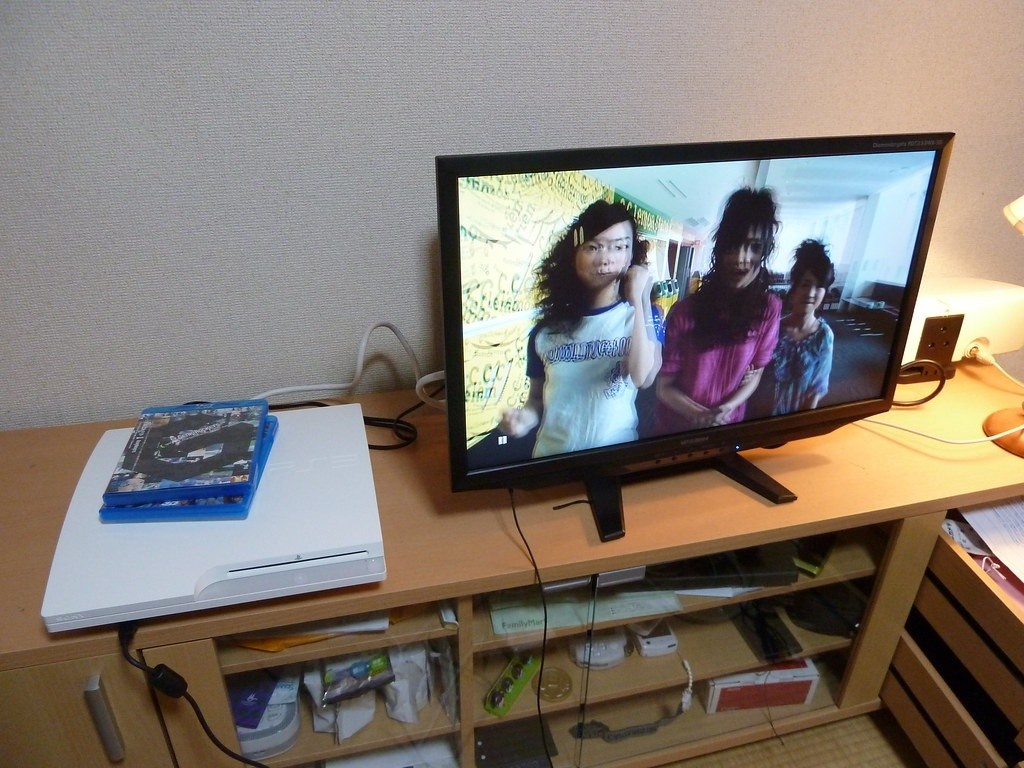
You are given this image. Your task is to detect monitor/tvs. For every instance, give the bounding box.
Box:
[435,131,954,493]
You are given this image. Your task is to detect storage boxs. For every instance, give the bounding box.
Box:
[692,656,821,715]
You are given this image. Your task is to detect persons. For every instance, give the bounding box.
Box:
[742,234,840,426]
[655,185,786,440]
[489,195,666,460]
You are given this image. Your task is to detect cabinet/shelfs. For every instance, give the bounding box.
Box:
[139,509,953,768]
[878,494,1024,768]
[1,648,176,767]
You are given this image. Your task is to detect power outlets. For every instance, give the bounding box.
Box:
[912,314,966,371]
